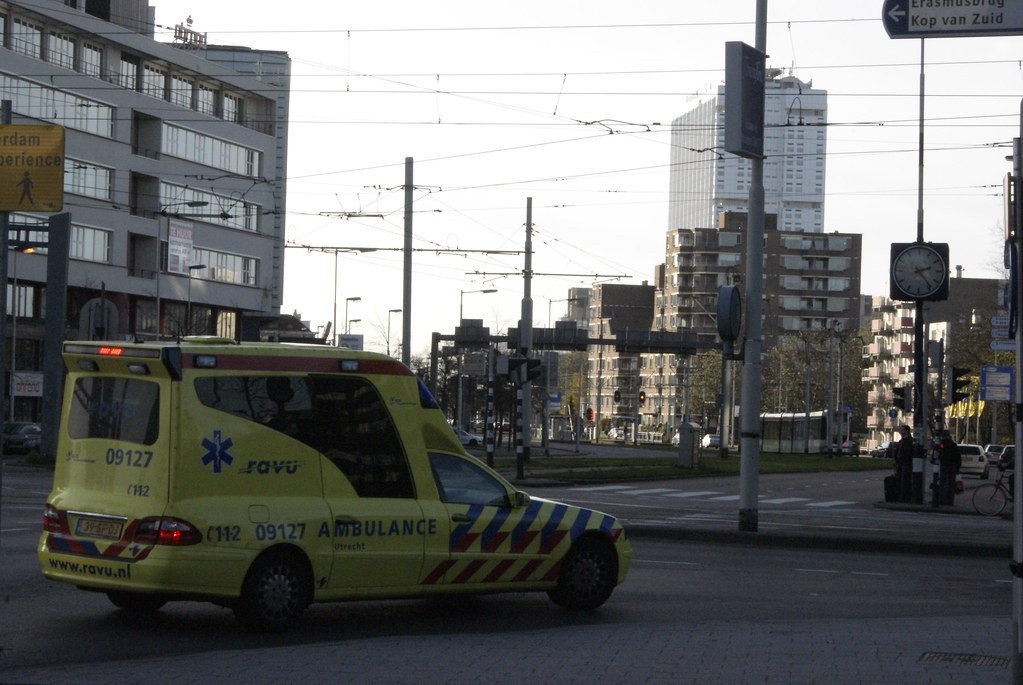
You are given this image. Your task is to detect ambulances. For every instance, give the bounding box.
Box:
[37,334,635,634]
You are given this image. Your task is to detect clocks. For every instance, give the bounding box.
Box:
[892,246,945,298]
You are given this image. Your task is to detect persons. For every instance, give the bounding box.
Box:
[624,425,627,440]
[466,419,470,433]
[604,424,609,434]
[453,420,457,432]
[938,430,962,505]
[1001,456,1015,502]
[894,425,913,502]
[487,411,495,431]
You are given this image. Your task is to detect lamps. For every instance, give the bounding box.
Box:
[160,201,209,208]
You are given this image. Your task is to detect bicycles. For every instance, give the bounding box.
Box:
[972,463,1014,517]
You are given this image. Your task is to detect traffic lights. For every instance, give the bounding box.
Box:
[585,390,646,421]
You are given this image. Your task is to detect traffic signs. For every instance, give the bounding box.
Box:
[881,0,1023,39]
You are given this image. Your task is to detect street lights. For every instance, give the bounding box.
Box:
[548,297,584,329]
[154,200,211,342]
[460,288,499,320]
[331,245,403,357]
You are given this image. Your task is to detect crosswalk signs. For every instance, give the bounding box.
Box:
[0,123,67,213]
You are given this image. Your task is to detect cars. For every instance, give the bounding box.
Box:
[985,443,1005,464]
[832,441,861,457]
[870,440,898,458]
[470,419,510,431]
[454,428,482,447]
[700,434,720,449]
[0,421,42,454]
[606,427,625,440]
[998,444,1015,472]
[672,432,680,445]
[956,444,989,480]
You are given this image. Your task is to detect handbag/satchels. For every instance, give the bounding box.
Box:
[955,474,964,494]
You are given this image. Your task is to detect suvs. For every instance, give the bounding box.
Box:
[860,446,868,455]
